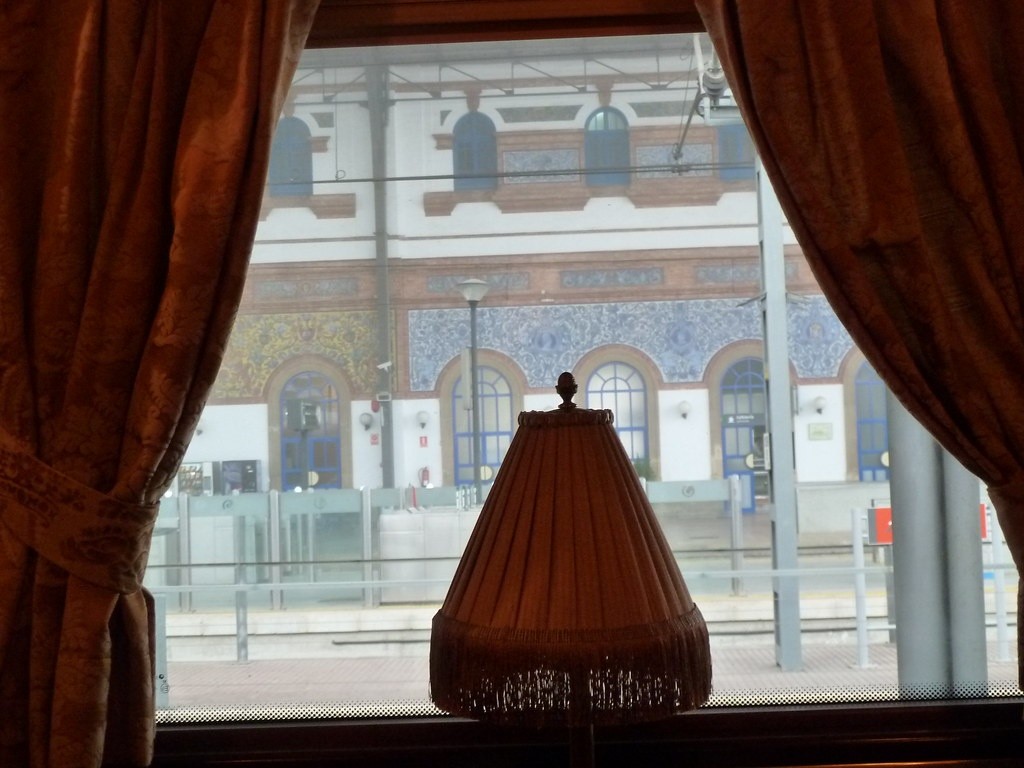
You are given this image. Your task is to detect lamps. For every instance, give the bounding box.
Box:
[427,371,714,767]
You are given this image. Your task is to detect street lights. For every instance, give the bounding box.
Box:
[453,276,490,510]
